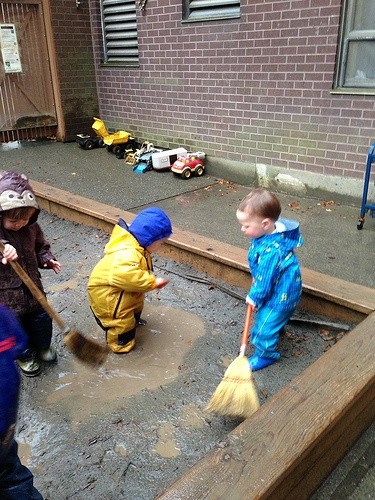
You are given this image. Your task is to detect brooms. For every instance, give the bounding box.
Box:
[207,306,259,419]
[1,241,108,370]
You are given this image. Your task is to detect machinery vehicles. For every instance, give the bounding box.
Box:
[103,130,135,156]
[76,117,111,149]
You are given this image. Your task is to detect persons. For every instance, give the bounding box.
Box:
[87,206,173,354]
[0,304,43,500]
[0,169,62,376]
[235,188,305,370]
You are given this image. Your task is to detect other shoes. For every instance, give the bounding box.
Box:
[16,346,57,377]
[279,328,286,336]
[249,354,277,372]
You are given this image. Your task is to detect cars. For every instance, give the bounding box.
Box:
[172,158,206,180]
